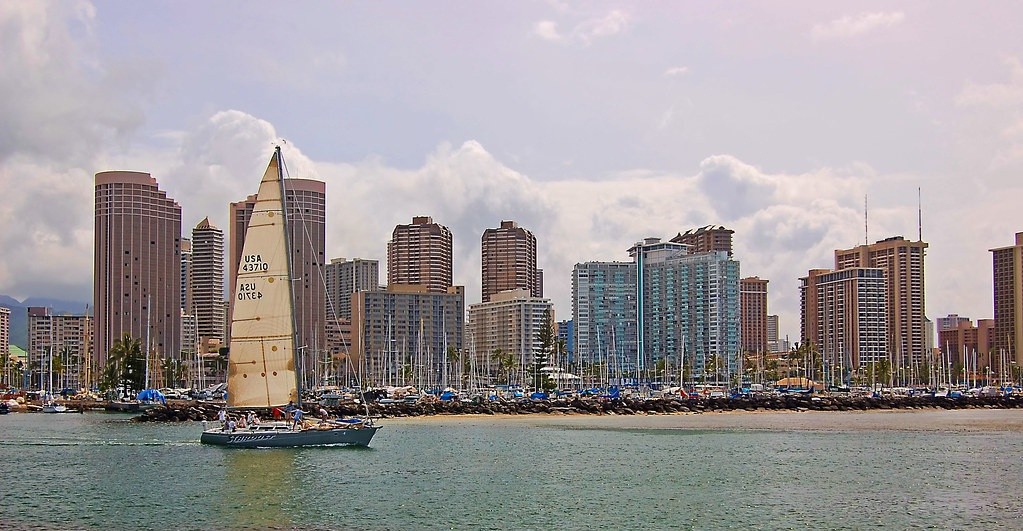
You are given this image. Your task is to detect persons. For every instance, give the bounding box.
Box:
[216,404,330,432]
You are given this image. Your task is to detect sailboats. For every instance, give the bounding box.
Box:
[200,138,383,448]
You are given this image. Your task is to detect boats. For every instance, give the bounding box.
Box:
[58,294,1022,417]
